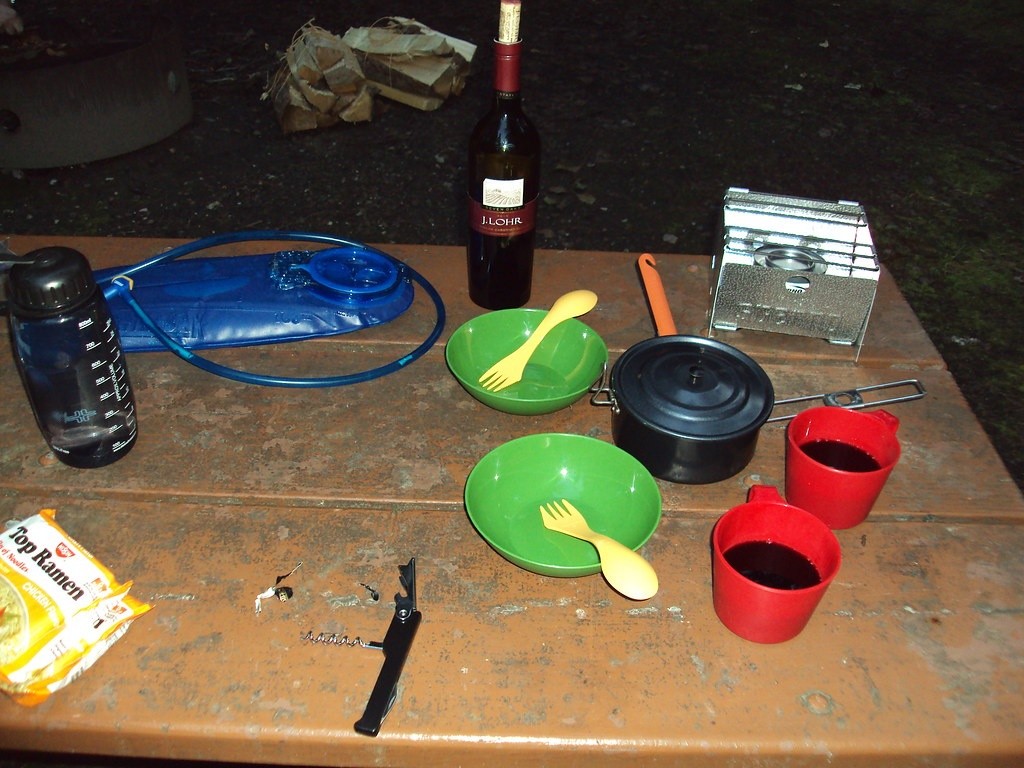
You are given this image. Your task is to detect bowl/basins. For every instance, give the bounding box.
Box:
[464,434,661,579]
[446,307,607,417]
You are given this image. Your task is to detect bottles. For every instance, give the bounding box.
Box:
[469,0,542,309]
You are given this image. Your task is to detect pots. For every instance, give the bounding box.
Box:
[594,335,775,488]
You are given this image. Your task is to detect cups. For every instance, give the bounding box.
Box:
[0,245,140,468]
[712,484,841,643]
[785,406,901,529]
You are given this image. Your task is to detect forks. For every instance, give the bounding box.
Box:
[479,288,597,392]
[539,498,661,600]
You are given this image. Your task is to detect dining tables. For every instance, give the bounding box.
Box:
[0,232,1024,768]
